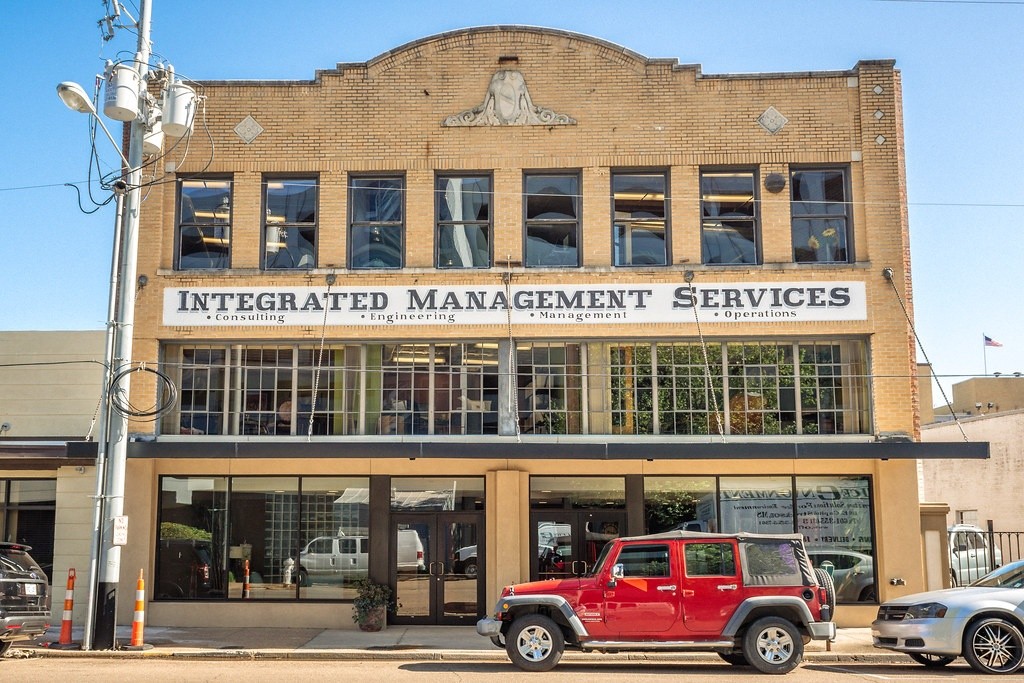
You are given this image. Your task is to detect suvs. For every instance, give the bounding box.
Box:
[539,532,618,582]
[0,542,51,655]
[476,530,835,673]
[154,537,214,600]
[454,523,588,580]
[947,524,1002,588]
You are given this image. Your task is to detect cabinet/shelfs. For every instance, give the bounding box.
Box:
[377,415,404,436]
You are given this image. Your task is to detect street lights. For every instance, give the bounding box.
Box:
[57,81,141,649]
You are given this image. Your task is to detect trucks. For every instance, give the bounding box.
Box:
[662,488,873,557]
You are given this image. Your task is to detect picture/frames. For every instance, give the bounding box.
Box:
[240,387,272,425]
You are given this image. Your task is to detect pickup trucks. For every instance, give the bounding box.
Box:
[397,529,426,572]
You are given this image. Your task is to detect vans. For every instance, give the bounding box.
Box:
[290,537,369,587]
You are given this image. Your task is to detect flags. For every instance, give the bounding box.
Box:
[985,336,1004,347]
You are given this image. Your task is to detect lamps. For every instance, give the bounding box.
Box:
[975,402,994,416]
[182,180,286,250]
[613,193,755,233]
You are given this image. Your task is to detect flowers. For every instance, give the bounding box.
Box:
[807,228,842,262]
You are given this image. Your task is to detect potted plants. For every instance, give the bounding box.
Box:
[158,521,212,598]
[351,578,403,632]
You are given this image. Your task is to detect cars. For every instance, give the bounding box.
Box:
[870,558,1024,674]
[803,546,875,600]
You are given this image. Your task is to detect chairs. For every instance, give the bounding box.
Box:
[704,391,767,435]
[417,404,445,436]
